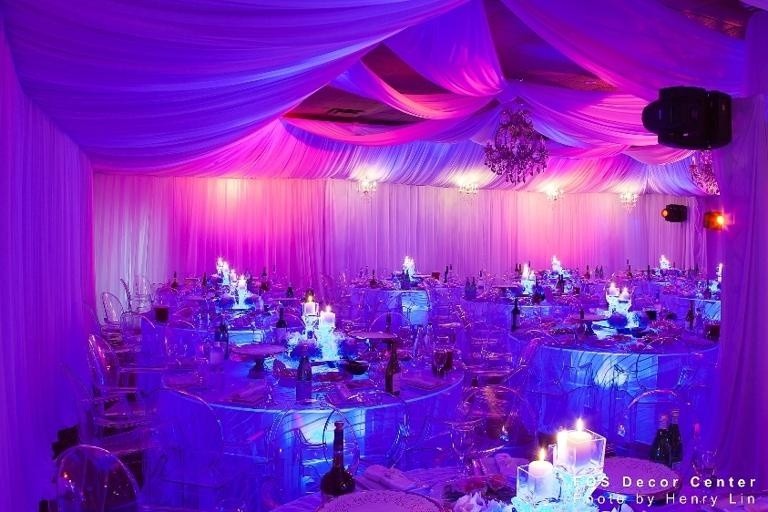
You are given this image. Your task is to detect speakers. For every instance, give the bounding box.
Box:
[642,85,733,150]
[664,204,687,222]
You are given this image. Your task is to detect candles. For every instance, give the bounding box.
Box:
[716,263,723,284]
[229,268,237,296]
[521,263,536,294]
[659,254,670,270]
[551,255,563,275]
[617,286,632,316]
[216,256,223,275]
[233,274,249,308]
[301,294,320,341]
[606,281,620,318]
[222,261,230,284]
[402,255,415,278]
[319,305,336,346]
[511,447,568,512]
[548,416,607,512]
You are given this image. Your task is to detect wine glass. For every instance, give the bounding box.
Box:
[575,322,587,350]
[692,443,717,502]
[431,347,447,379]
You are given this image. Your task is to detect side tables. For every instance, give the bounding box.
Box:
[268,465,767,512]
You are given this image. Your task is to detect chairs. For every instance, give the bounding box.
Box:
[52,444,144,512]
[80,333,145,457]
[144,387,265,512]
[100,275,203,368]
[369,311,693,438]
[265,384,701,505]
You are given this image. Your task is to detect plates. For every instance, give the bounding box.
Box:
[431,477,515,507]
[593,455,682,503]
[316,489,444,512]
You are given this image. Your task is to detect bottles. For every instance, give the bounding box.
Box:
[465,277,477,298]
[444,264,454,283]
[510,298,521,333]
[704,279,711,299]
[575,309,588,343]
[385,340,402,397]
[423,322,435,360]
[295,344,313,405]
[412,324,426,367]
[647,416,676,508]
[321,420,356,496]
[685,300,696,335]
[594,266,604,281]
[583,266,590,281]
[627,265,632,279]
[370,270,377,289]
[261,267,267,280]
[213,313,228,363]
[646,265,652,280]
[558,274,565,295]
[171,272,178,292]
[398,269,410,291]
[363,266,370,282]
[274,308,287,344]
[201,272,207,287]
[669,410,683,464]
[515,264,521,280]
[286,282,294,298]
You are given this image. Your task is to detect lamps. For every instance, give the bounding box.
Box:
[458,182,479,201]
[620,192,641,213]
[660,205,685,223]
[544,189,564,204]
[356,178,378,202]
[700,210,724,232]
[483,108,550,187]
